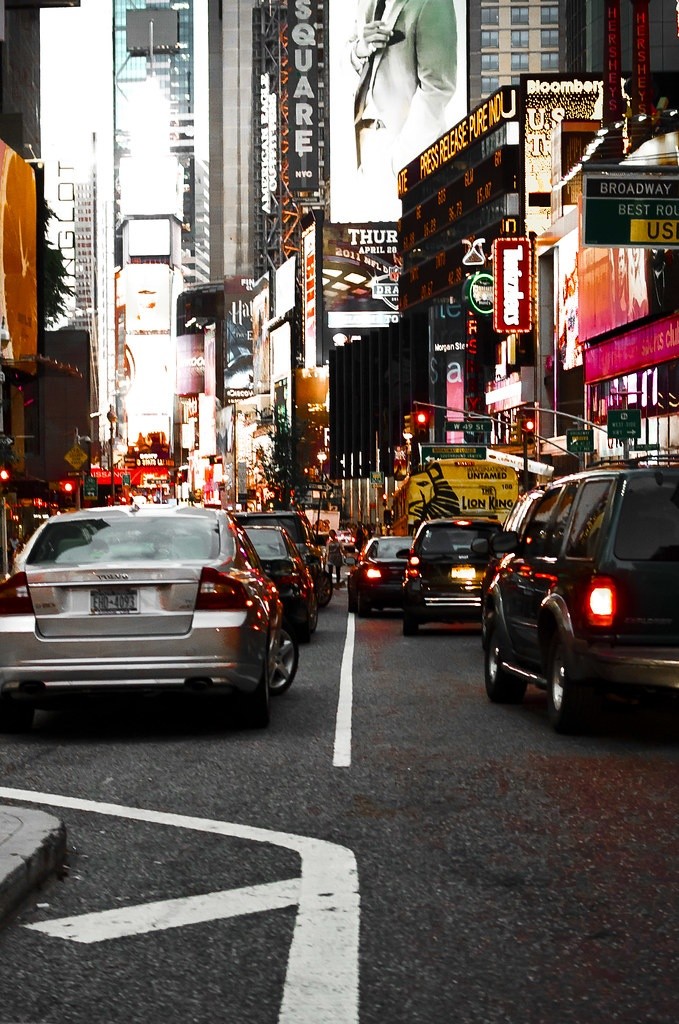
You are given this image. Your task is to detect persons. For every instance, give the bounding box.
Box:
[343,0,458,169]
[326,529,344,591]
[347,522,391,552]
[8,532,19,551]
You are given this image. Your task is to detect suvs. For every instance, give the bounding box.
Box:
[231,512,335,611]
[396,516,499,638]
[465,451,679,736]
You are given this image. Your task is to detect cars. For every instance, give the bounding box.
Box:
[241,525,320,640]
[342,533,414,619]
[0,504,300,731]
